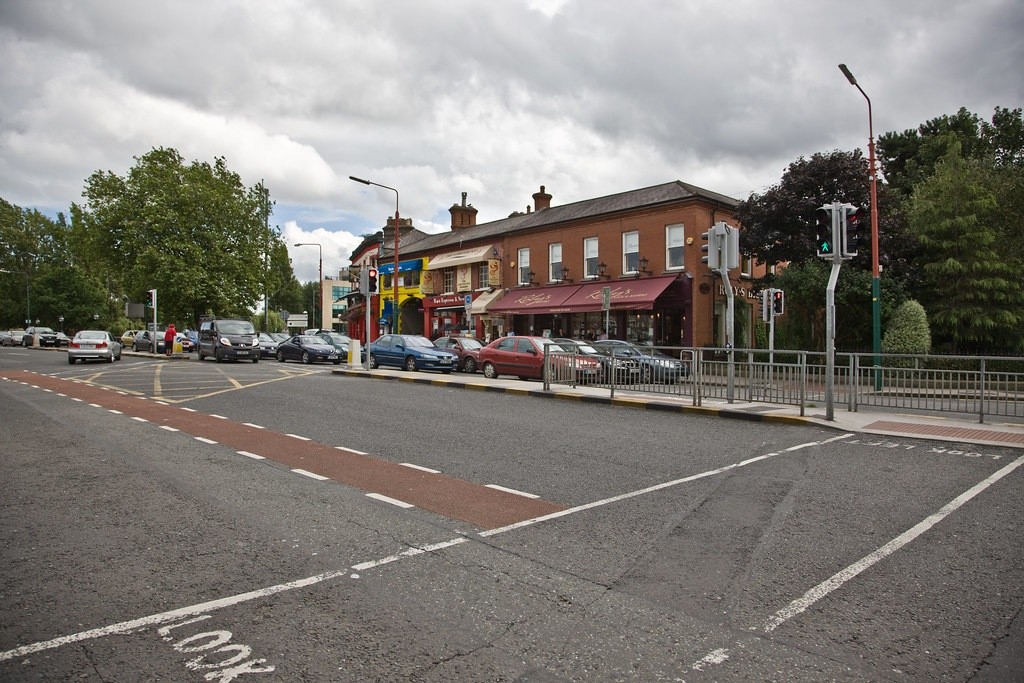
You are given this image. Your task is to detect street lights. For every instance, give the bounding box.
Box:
[337,313,341,332]
[349,175,399,335]
[294,243,322,332]
[58,314,65,332]
[837,64,883,391]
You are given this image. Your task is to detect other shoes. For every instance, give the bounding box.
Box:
[167,354,171,356]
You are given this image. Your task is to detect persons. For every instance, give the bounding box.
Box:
[587,327,597,340]
[555,328,565,337]
[507,327,515,336]
[600,329,606,340]
[430,329,439,341]
[164,324,177,356]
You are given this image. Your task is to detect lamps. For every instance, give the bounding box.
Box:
[560,266,573,283]
[639,256,652,276]
[597,261,611,281]
[527,269,539,286]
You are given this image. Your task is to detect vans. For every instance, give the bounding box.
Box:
[197,319,260,363]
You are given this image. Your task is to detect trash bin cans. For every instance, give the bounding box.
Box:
[32,334,41,347]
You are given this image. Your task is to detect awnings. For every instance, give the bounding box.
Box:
[471,289,505,313]
[379,259,422,274]
[486,273,677,315]
[427,245,495,270]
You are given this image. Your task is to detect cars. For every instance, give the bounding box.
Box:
[477,336,689,381]
[433,336,484,373]
[68,330,122,364]
[361,334,459,373]
[120,330,200,354]
[258,329,364,365]
[0,325,70,348]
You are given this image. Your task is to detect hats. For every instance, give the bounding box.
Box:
[170,324,174,328]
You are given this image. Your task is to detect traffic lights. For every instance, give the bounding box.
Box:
[812,205,834,256]
[842,204,866,257]
[701,228,720,268]
[757,291,768,322]
[358,269,368,294]
[773,290,784,314]
[146,290,154,308]
[368,268,379,294]
